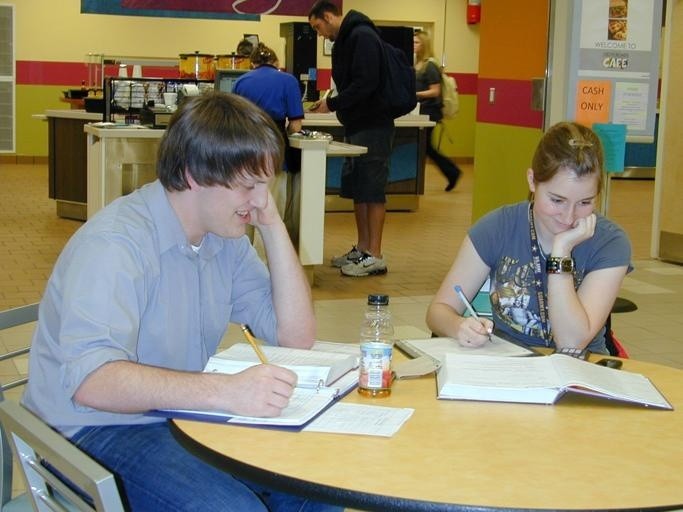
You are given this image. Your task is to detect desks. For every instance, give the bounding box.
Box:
[31,94,436,288]
[167,345,683,511]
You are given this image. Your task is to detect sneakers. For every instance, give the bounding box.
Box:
[340,249,387,277]
[331,245,362,267]
[445,168,461,192]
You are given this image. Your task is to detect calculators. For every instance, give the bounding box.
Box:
[553,347,588,361]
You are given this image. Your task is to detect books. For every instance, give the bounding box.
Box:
[142,339,360,433]
[434,352,673,411]
[392,328,544,362]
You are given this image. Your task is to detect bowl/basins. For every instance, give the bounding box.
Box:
[62,90,104,113]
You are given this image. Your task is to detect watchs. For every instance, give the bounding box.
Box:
[546,256,575,275]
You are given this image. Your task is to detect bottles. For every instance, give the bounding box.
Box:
[117,65,128,78]
[359,294,391,396]
[81,80,86,91]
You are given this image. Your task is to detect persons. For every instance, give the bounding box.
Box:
[18,87,344,511]
[413,30,463,192]
[308,1,396,278]
[424,120,635,355]
[231,44,305,261]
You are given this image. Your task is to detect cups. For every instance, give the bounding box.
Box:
[131,65,142,78]
[163,93,178,107]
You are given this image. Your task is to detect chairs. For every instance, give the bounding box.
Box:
[431,276,637,357]
[0,302,92,512]
[0,400,126,511]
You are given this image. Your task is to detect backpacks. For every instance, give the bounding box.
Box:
[428,57,459,119]
[335,20,417,119]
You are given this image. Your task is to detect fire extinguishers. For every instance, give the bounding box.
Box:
[467,0,481,24]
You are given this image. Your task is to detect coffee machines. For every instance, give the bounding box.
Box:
[276,23,322,101]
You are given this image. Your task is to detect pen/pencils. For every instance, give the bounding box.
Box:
[455,285,493,343]
[240,324,267,364]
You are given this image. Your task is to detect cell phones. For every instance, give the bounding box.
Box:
[594,358,624,369]
